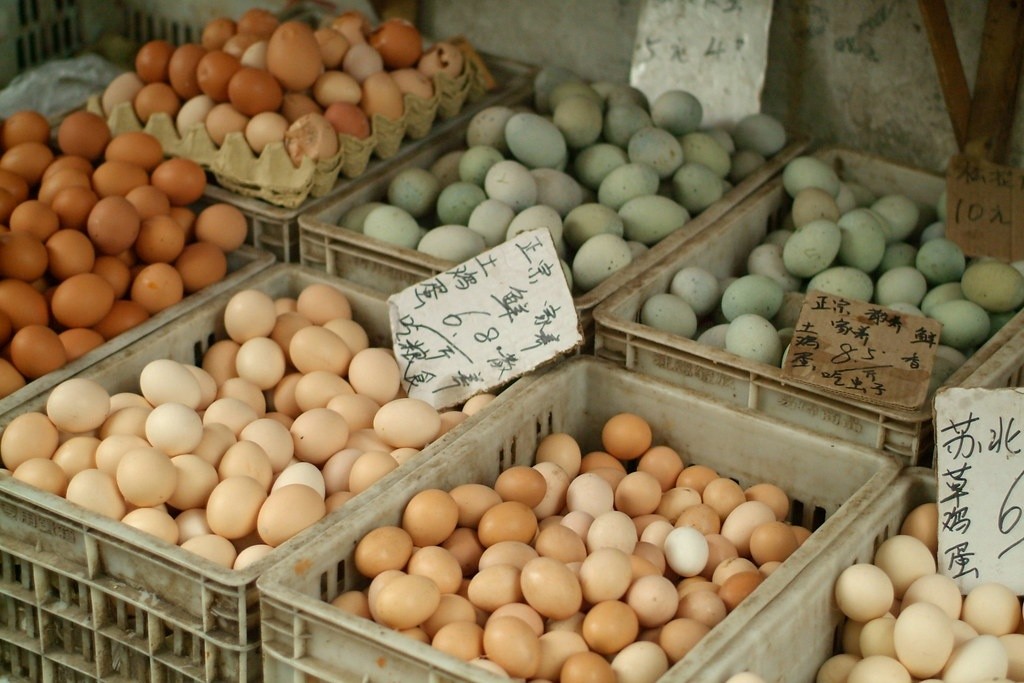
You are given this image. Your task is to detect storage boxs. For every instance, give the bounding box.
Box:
[2,1,1024,682]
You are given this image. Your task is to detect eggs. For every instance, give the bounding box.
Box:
[0,110,248,399]
[102,8,468,166]
[335,75,786,296]
[720,499,1024,683]
[0,283,497,572]
[639,154,1024,406]
[324,410,817,683]
